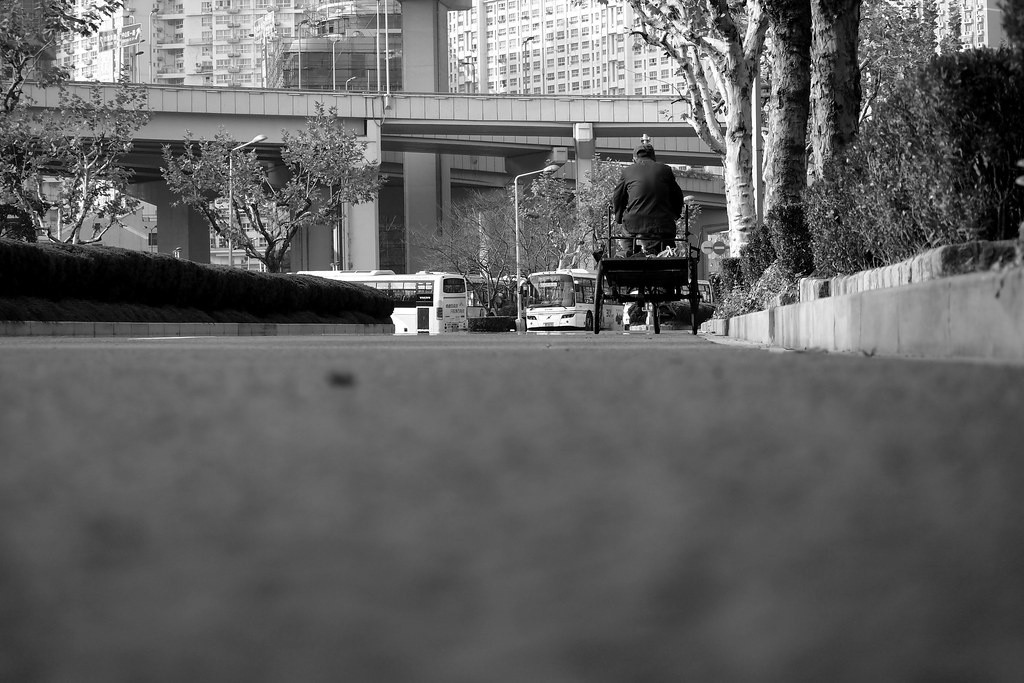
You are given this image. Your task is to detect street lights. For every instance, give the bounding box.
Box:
[298,20,308,89]
[149,8,159,84]
[525,37,534,94]
[144,225,157,253]
[333,40,340,91]
[346,77,356,91]
[134,51,144,83]
[229,134,269,267]
[248,237,258,270]
[462,63,475,93]
[137,39,145,82]
[515,165,560,332]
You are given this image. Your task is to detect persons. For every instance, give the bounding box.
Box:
[612,144,684,256]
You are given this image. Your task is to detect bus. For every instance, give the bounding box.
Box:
[416,271,528,318]
[518,269,630,331]
[675,279,713,304]
[287,269,468,334]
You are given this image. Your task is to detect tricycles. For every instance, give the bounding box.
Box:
[593,204,700,335]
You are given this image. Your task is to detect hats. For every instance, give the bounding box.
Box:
[633,143,655,159]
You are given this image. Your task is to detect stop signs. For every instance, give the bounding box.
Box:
[713,242,725,255]
